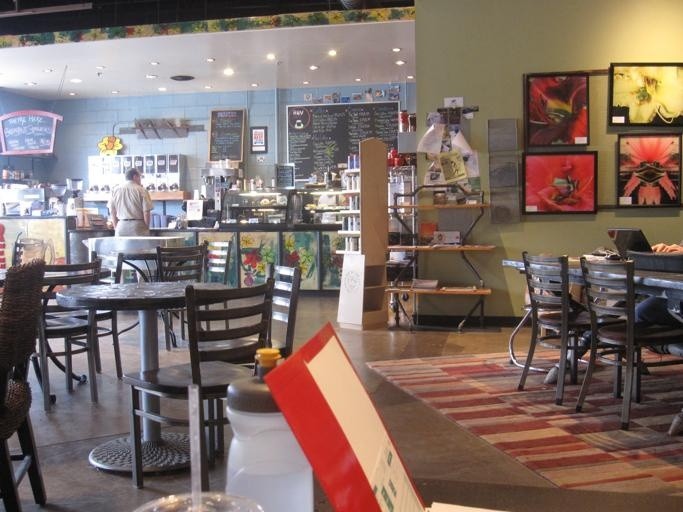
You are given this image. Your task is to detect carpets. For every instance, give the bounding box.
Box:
[366,348,683,496]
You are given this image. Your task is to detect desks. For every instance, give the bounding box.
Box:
[66,223,343,297]
[502,256,683,436]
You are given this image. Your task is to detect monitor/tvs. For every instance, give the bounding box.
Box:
[607,227,654,261]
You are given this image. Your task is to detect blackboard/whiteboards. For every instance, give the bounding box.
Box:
[274,163,295,190]
[286,100,401,181]
[208,107,245,163]
[0,109,63,156]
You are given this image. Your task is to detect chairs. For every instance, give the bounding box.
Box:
[38,251,101,411]
[518,252,625,406]
[0,259,46,511]
[181,240,233,340]
[66,253,125,393]
[187,262,302,457]
[156,243,211,351]
[575,257,683,431]
[122,279,274,489]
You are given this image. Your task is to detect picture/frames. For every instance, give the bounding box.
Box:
[250,126,268,154]
[617,133,681,207]
[523,71,589,148]
[609,62,683,128]
[522,151,598,214]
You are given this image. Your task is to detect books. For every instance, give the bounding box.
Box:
[265,322,431,512]
[411,280,440,292]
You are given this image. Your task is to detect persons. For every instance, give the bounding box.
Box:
[632,240,683,321]
[106,168,154,236]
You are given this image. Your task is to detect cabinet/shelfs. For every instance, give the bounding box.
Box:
[1,217,77,299]
[386,184,497,334]
[219,189,303,224]
[335,168,365,331]
[305,184,350,221]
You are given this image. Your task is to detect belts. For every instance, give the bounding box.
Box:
[119,218,139,222]
